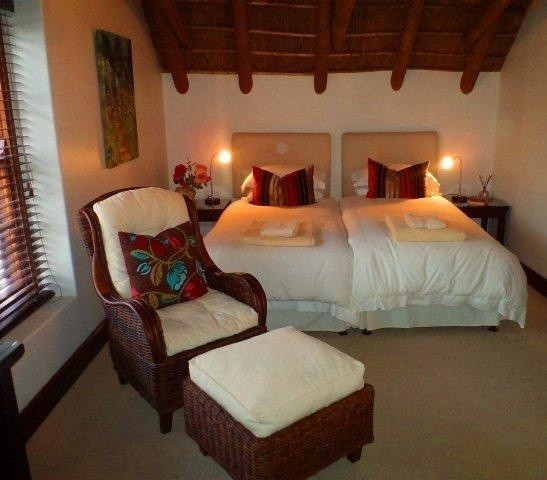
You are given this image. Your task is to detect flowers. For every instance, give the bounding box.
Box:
[170,161,211,190]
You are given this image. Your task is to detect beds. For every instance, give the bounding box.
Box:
[204,132,526,334]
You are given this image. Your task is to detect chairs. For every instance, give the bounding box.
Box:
[76,186,268,434]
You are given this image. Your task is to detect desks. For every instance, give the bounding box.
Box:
[196,198,231,222]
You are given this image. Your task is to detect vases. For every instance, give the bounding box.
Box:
[173,184,196,199]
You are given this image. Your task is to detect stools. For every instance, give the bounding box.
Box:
[182,326,374,480]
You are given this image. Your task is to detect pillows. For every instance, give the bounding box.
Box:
[117,221,208,311]
